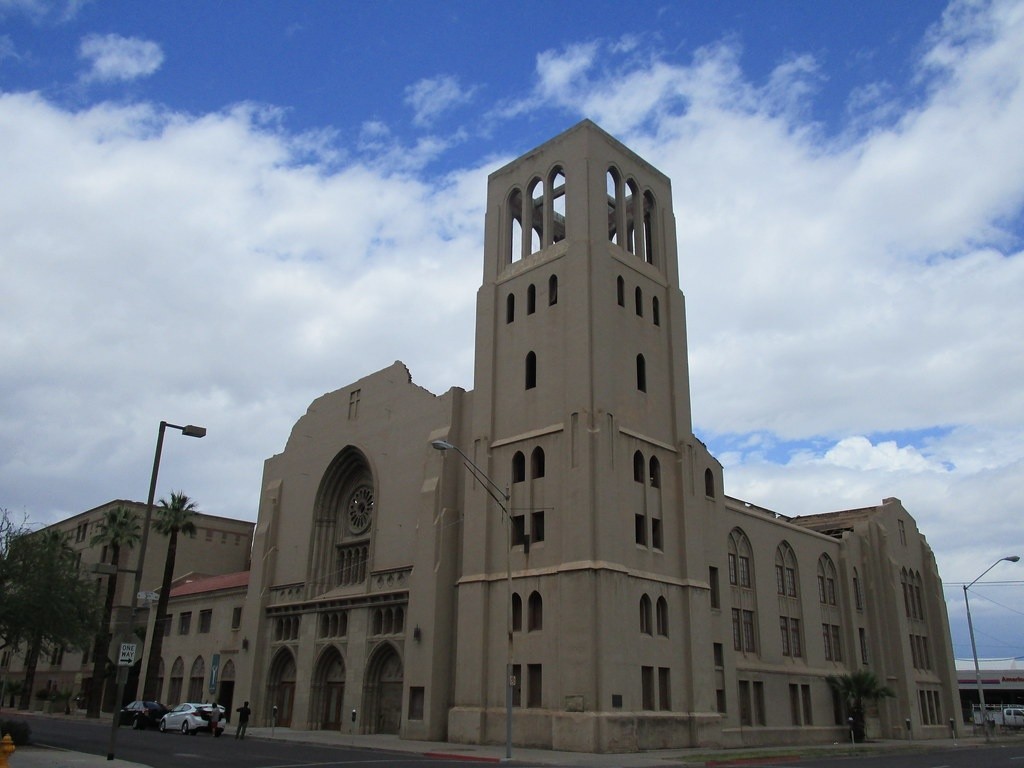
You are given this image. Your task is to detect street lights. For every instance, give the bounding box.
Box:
[426,436,517,759]
[105,420,209,761]
[961,554,1021,741]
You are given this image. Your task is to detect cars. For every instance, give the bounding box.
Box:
[159,702,227,737]
[118,700,169,730]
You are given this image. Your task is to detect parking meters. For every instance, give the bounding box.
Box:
[949,717,957,747]
[905,717,912,747]
[848,717,855,749]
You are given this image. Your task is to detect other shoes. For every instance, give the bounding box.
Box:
[234,737,245,740]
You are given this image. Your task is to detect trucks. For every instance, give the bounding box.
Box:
[971,703,1024,727]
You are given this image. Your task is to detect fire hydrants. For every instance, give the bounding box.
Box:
[0,733,15,768]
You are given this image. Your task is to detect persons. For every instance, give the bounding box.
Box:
[207,703,220,737]
[235,700,251,740]
